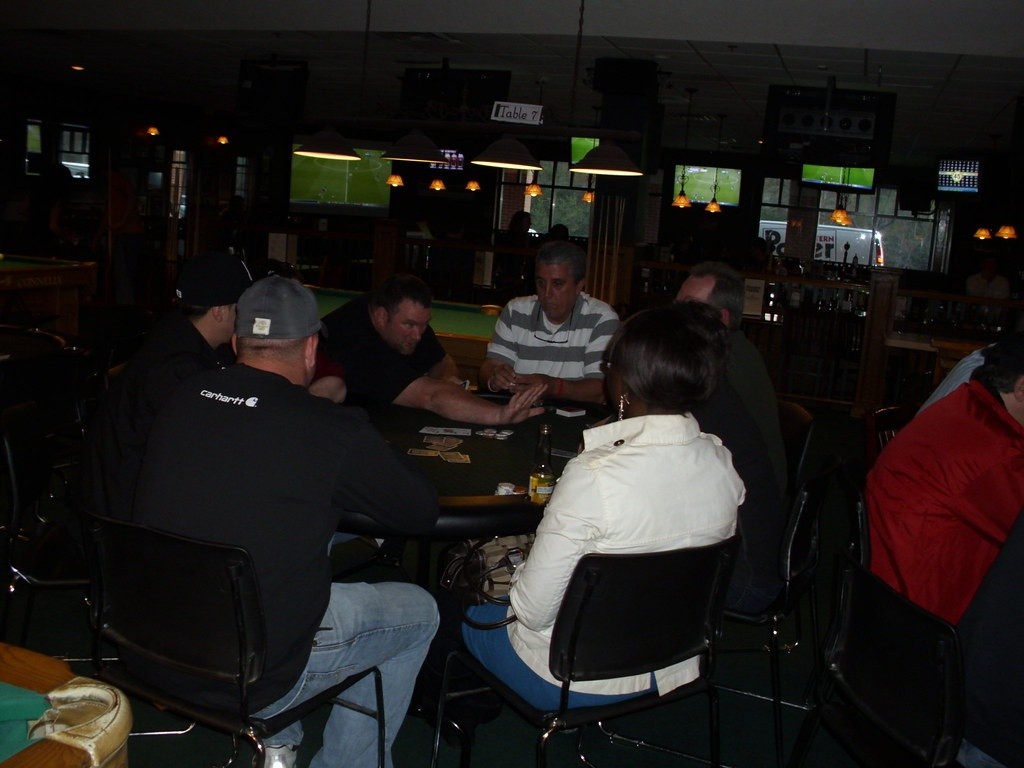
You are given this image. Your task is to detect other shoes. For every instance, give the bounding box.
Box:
[406,692,506,725]
[261,733,325,768]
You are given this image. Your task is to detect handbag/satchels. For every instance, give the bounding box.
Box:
[438,530,537,629]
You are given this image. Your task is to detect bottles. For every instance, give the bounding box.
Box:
[527,424,556,506]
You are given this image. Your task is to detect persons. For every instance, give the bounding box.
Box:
[970,256,1010,325]
[462,301,747,713]
[950,502,1024,768]
[507,209,532,245]
[243,257,347,403]
[671,262,788,611]
[101,251,248,523]
[23,164,66,250]
[477,240,620,405]
[317,275,549,425]
[92,162,147,270]
[219,196,244,225]
[135,276,440,768]
[865,331,1024,623]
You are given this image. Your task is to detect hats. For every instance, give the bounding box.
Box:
[235,275,329,340]
[175,262,242,306]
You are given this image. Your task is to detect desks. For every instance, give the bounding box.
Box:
[336,391,627,587]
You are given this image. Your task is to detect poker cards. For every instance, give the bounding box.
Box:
[550,447,578,459]
[556,406,586,418]
[406,426,472,464]
[459,380,470,391]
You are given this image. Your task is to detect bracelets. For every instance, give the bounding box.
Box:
[487,380,498,392]
[553,378,564,397]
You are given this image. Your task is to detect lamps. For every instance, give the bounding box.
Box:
[673,86,700,207]
[292,116,646,204]
[832,181,855,228]
[971,134,1016,240]
[705,112,728,214]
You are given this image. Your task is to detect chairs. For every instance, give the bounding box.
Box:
[434,399,964,768]
[1,305,385,767]
